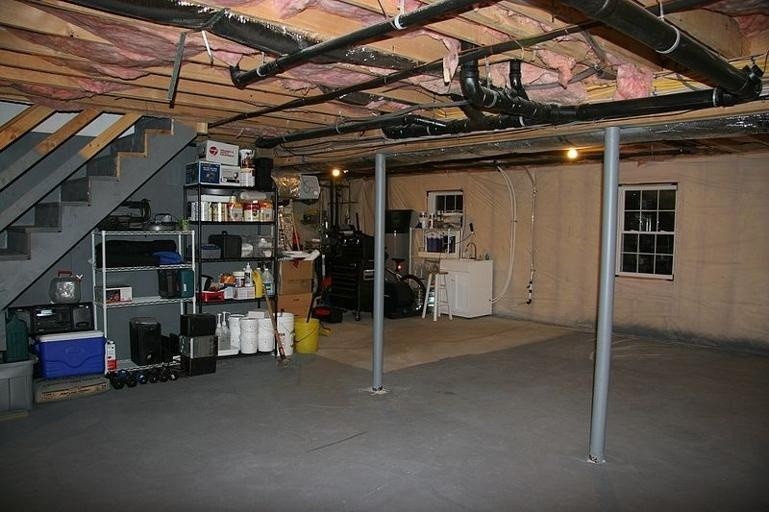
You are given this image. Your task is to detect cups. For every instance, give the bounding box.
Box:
[180,219,188,231]
[233,272,244,288]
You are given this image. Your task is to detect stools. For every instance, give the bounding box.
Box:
[422,270,453,322]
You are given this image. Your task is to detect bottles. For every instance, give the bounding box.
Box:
[324,216,328,230]
[107,340,117,371]
[256,267,275,296]
[418,209,443,230]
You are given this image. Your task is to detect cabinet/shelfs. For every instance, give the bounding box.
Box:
[413,227,463,259]
[438,271,469,319]
[183,182,278,358]
[91,230,197,373]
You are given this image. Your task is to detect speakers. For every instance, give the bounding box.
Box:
[130,319,161,365]
[180,314,216,376]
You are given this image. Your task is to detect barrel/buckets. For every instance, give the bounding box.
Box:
[261,268,275,295]
[227,309,320,357]
[5,313,29,362]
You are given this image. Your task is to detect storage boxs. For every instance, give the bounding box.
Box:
[185,161,221,183]
[240,168,255,187]
[39,329,107,380]
[276,260,316,317]
[0,349,39,413]
[196,139,239,166]
[208,234,242,259]
[234,286,256,300]
[221,164,241,186]
[93,285,133,304]
[179,313,218,376]
[187,243,221,259]
[156,269,194,299]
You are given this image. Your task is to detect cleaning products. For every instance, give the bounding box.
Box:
[485,253,489,260]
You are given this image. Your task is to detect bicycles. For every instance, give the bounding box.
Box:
[384,247,427,316]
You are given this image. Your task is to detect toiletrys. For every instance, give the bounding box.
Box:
[215,310,231,350]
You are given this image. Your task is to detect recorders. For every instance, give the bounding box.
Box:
[30,302,94,335]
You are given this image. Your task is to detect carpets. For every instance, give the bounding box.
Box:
[317,308,575,373]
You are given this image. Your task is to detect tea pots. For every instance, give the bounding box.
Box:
[239,150,253,168]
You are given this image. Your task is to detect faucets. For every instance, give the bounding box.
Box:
[467,242,477,259]
